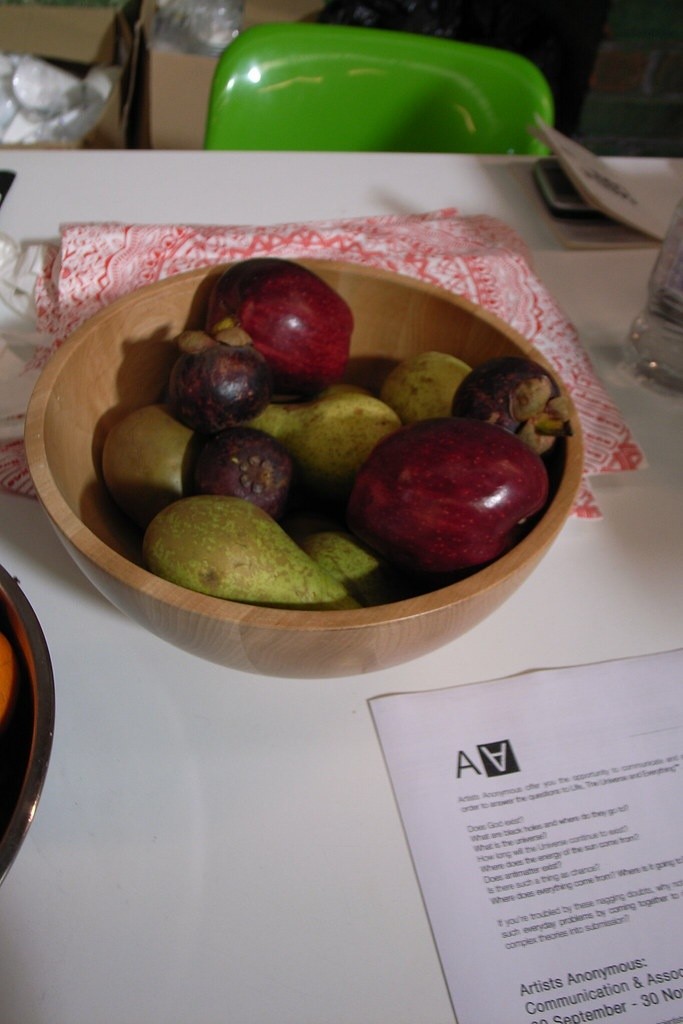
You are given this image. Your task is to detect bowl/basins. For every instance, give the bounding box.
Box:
[0,564,55,887]
[23,256,586,681]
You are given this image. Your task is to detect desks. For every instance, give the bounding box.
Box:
[0,150,683,1024]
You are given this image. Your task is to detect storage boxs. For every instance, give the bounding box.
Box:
[0,5,141,150]
[141,1,330,152]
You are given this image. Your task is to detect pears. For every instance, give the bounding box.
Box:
[101,351,473,610]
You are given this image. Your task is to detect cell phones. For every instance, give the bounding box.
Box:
[532,156,605,221]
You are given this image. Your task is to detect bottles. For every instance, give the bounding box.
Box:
[628,193,683,397]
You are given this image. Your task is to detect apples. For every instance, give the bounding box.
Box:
[203,258,354,394]
[350,417,549,584]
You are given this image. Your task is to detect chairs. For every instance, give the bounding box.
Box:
[201,19,554,152]
